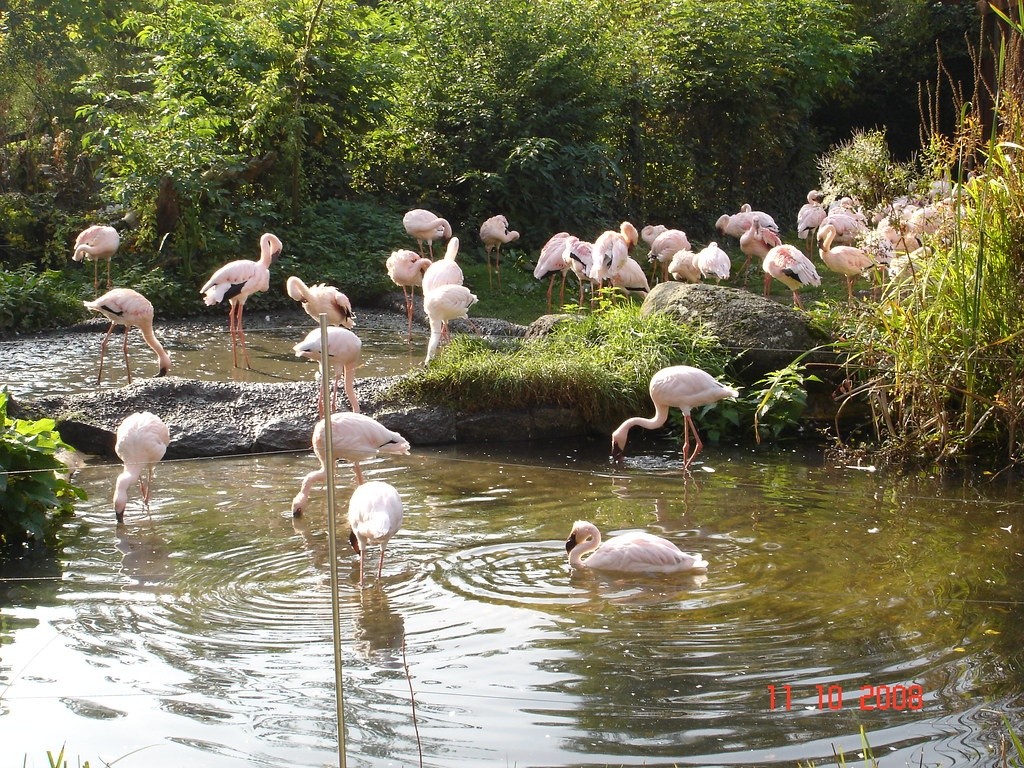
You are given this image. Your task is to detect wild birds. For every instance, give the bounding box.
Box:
[72,224,119,300]
[611,363,745,470]
[113,410,172,527]
[740,216,782,301]
[532,221,733,316]
[292,324,362,418]
[795,180,965,306]
[715,210,780,291]
[291,411,411,520]
[761,244,822,311]
[286,273,357,329]
[386,209,480,369]
[346,481,404,586]
[199,232,283,373]
[480,214,520,292]
[566,516,710,572]
[82,287,172,387]
